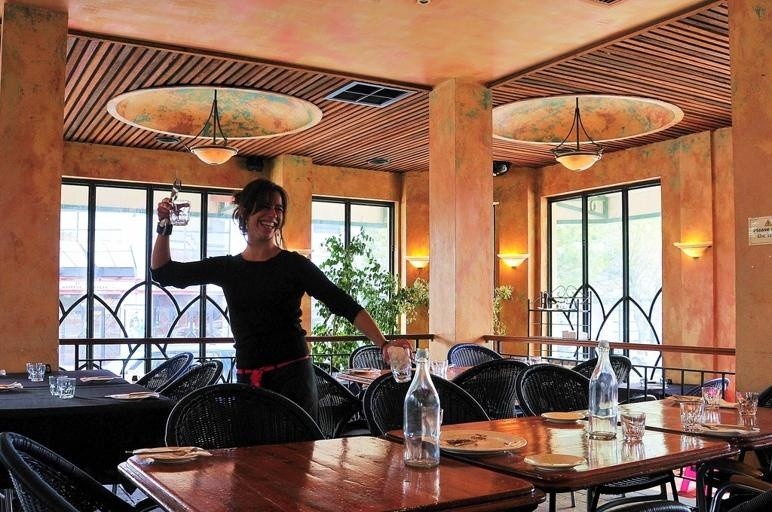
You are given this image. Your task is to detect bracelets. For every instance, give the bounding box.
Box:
[157,218,173,235]
[380,339,389,354]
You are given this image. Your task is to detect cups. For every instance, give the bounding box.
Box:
[681,387,759,432]
[431,359,448,378]
[517,356,541,365]
[49,376,76,398]
[26,362,46,382]
[640,377,669,387]
[619,411,646,443]
[170,200,191,226]
[387,346,411,383]
[562,331,587,340]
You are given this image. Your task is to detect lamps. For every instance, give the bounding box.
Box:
[496,253,530,269]
[671,241,713,258]
[549,96,607,174]
[401,255,430,269]
[183,90,239,167]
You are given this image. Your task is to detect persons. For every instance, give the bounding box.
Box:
[151,179,413,443]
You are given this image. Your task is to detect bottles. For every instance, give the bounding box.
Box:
[556,301,567,310]
[403,341,440,468]
[589,340,618,440]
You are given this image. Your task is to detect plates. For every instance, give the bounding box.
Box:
[542,409,628,423]
[666,396,738,409]
[524,455,587,470]
[699,423,760,437]
[438,429,527,455]
[0,383,23,392]
[347,368,381,374]
[80,376,114,384]
[133,448,203,463]
[105,392,160,402]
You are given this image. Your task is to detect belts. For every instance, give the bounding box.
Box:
[236,353,313,387]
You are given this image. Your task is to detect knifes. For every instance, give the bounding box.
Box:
[126,448,203,454]
